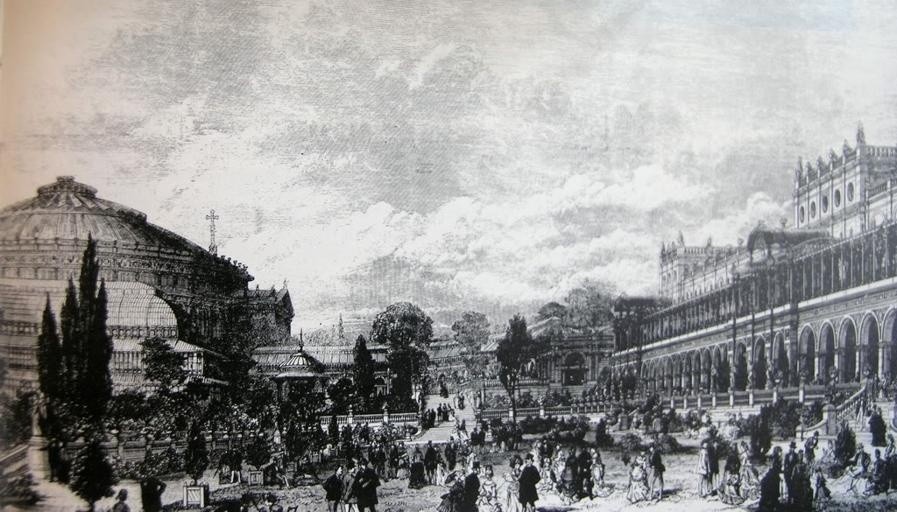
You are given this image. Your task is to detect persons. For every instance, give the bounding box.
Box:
[38,366,896,512]
